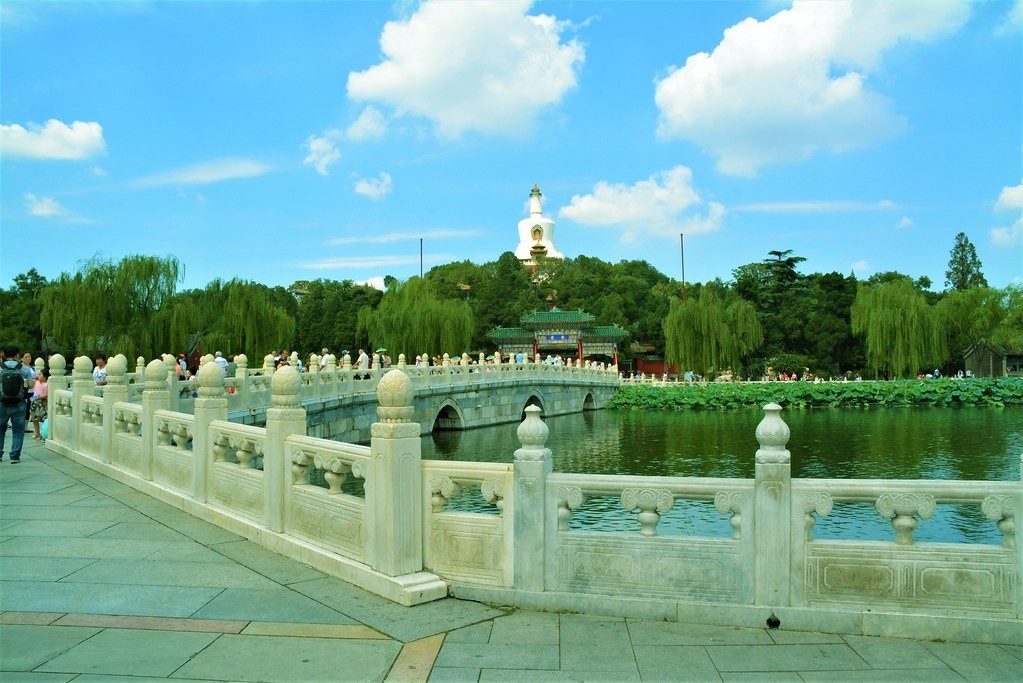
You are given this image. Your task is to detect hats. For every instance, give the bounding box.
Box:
[180,354,186,359]
[291,351,298,355]
[416,356,422,362]
[342,350,349,354]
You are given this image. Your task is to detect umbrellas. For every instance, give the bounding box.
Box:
[486,355,494,360]
[450,356,461,363]
[376,347,387,352]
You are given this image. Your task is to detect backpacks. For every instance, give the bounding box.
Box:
[0,362,23,398]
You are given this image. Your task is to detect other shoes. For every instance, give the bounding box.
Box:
[30,435,42,440]
[7,425,12,428]
[9,452,20,464]
[24,430,33,433]
[0,451,4,460]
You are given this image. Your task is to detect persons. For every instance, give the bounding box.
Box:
[0,347,70,443]
[762,370,861,382]
[884,371,889,381]
[93,353,108,397]
[660,372,669,382]
[690,372,733,383]
[1006,365,1023,372]
[589,362,608,374]
[516,348,574,371]
[917,368,941,380]
[958,368,972,380]
[263,348,391,385]
[176,351,238,399]
[635,370,641,379]
[415,355,497,376]
[0,345,36,463]
